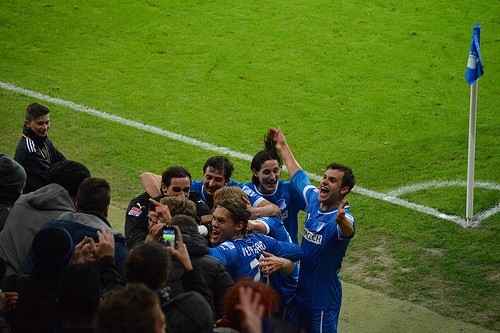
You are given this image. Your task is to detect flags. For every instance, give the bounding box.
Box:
[465,30,484,86]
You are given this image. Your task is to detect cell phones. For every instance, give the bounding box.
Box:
[160,225,177,249]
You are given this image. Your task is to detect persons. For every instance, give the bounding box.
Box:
[246,149,307,245]
[139,156,281,218]
[14,102,67,194]
[93,284,166,333]
[124,222,215,308]
[213,278,279,333]
[214,186,299,281]
[125,166,197,250]
[0,154,27,232]
[0,160,91,276]
[43,177,130,275]
[165,215,233,321]
[269,126,356,333]
[204,199,303,285]
[6,225,126,333]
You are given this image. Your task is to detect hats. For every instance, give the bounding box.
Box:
[45,160,91,196]
[0,153,27,192]
[31,227,75,273]
